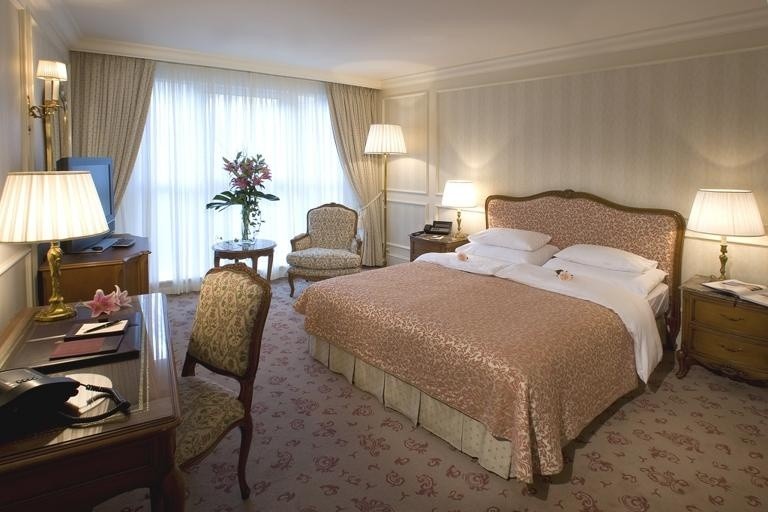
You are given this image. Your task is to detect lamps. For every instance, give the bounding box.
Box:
[363,124,408,267]
[441,180,479,239]
[687,188,766,282]
[29,60,68,116]
[0,171,110,322]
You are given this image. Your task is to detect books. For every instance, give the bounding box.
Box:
[62,320,130,342]
[699,279,768,307]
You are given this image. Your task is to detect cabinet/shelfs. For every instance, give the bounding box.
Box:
[38,234,152,307]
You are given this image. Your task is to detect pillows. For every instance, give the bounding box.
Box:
[455,228,669,300]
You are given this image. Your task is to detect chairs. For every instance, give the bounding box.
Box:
[175,263,272,499]
[287,203,362,297]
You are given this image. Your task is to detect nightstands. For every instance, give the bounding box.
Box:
[676,274,768,388]
[410,232,470,262]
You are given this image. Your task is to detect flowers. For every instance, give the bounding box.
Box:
[206,151,280,247]
[83,284,133,317]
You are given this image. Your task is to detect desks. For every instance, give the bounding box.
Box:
[0,294,184,512]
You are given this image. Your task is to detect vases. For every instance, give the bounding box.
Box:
[240,202,261,245]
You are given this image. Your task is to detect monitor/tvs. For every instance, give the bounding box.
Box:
[55,157,115,252]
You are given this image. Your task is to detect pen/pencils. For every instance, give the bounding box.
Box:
[84,319,122,333]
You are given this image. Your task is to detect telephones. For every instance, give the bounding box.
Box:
[412,221,452,236]
[0,367,131,440]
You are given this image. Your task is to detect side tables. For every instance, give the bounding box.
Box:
[213,240,277,281]
